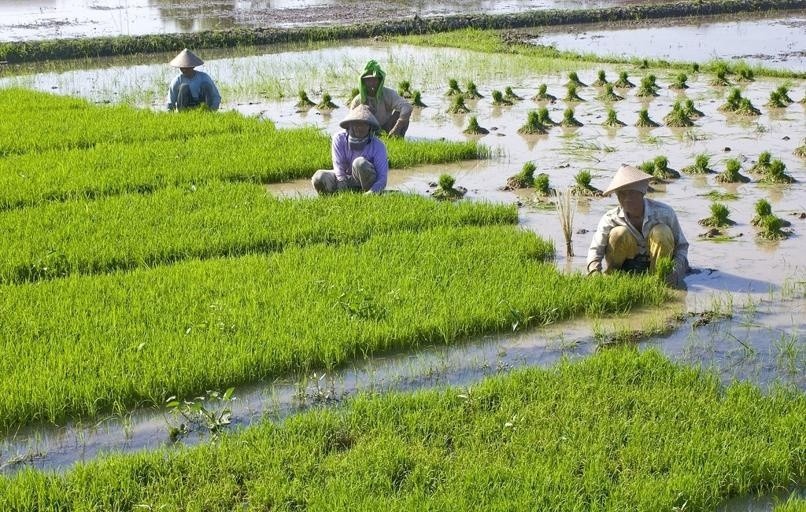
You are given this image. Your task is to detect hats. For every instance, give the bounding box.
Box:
[362,71,381,78]
[169,49,204,67]
[340,105,380,130]
[602,163,655,196]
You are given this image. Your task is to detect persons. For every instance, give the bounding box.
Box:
[348,60,412,136]
[587,167,689,285]
[312,104,388,195]
[167,49,220,110]
